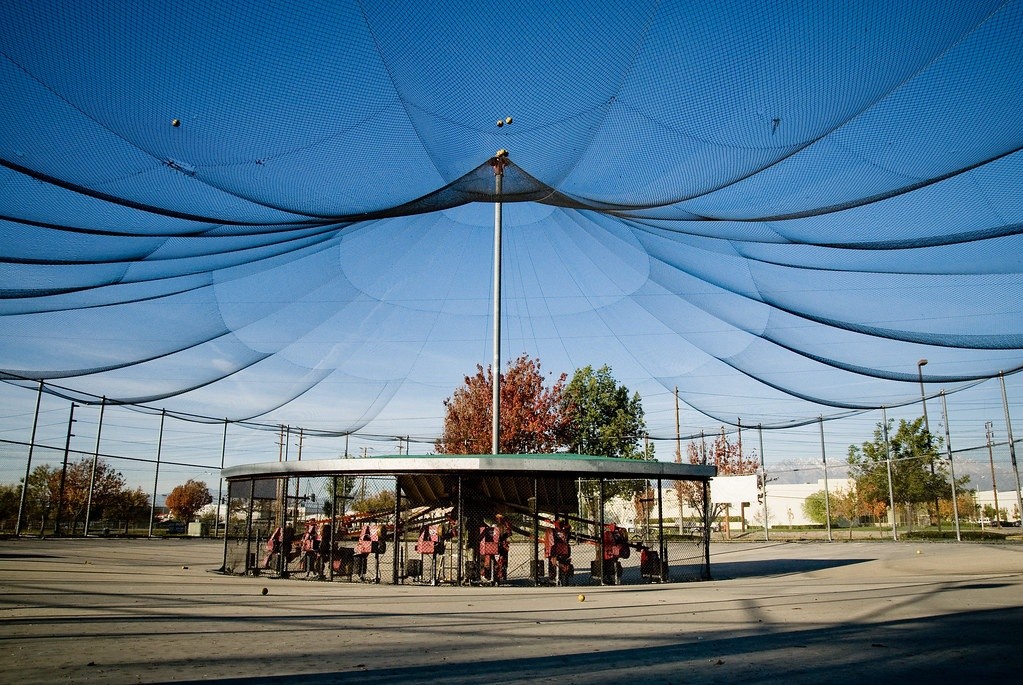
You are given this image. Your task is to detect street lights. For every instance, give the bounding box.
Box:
[917,359,943,536]
[359,446,374,528]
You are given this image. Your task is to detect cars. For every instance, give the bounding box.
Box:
[156,513,174,523]
[211,523,225,532]
[980,518,991,523]
